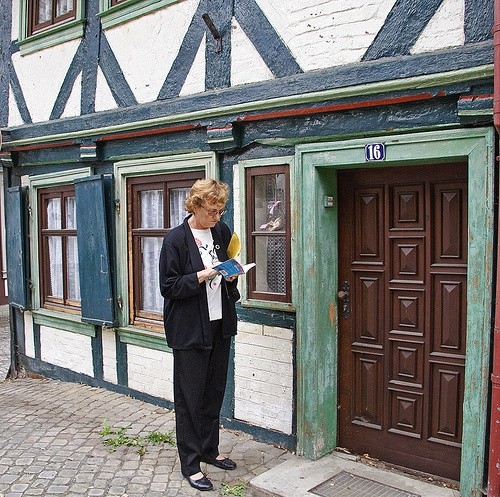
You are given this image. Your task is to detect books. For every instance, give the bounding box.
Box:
[212,258,257,278]
[226,231,242,259]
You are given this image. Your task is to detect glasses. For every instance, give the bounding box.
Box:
[199,203,228,216]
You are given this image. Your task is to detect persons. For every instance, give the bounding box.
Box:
[158,178,241,492]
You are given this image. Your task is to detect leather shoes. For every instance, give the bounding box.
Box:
[183,471,213,491]
[201,455,237,470]
